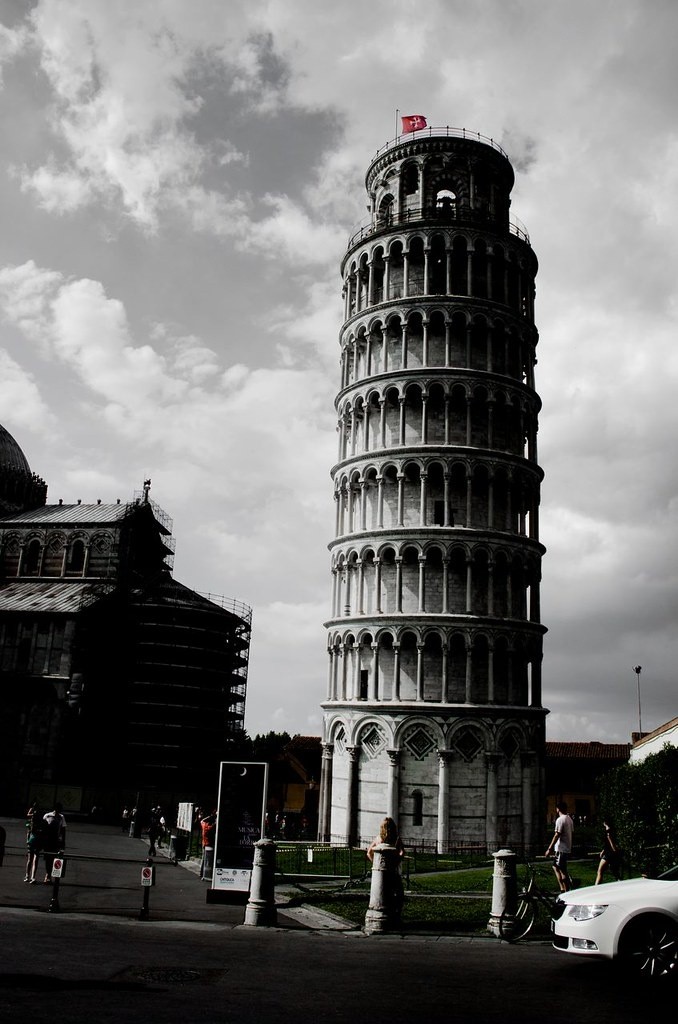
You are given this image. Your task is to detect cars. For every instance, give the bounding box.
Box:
[549,863,677,983]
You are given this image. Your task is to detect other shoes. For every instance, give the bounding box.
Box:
[29,879,36,884]
[43,878,50,883]
[24,878,29,882]
[159,845,164,848]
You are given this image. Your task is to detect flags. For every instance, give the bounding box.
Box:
[402,116,427,133]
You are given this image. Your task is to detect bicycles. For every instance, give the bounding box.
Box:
[498,860,574,945]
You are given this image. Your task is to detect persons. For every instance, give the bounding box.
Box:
[199,814,217,877]
[545,801,574,893]
[151,806,161,824]
[595,817,621,885]
[149,817,167,856]
[42,803,67,884]
[366,817,404,924]
[128,804,138,818]
[301,816,308,833]
[121,805,130,832]
[24,803,49,883]
[265,813,287,834]
